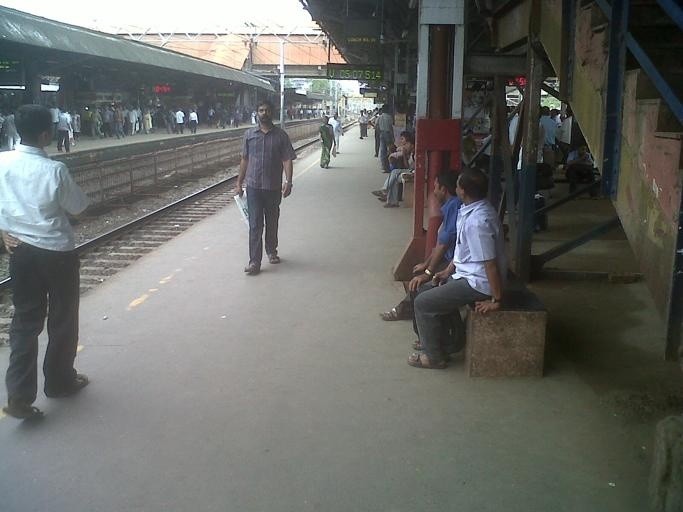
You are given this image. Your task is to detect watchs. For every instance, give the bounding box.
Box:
[284,180,292,186]
[423,267,431,278]
[489,296,502,303]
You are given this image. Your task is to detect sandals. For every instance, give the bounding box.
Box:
[45,374,88,397]
[408,339,447,369]
[4,405,43,418]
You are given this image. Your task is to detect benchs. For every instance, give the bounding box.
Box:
[463,261,551,382]
[400,175,428,209]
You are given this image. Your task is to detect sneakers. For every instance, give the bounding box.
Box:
[244,260,259,273]
[382,307,400,321]
[267,252,280,263]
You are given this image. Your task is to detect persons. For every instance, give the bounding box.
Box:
[83,101,200,138]
[328,113,342,155]
[357,107,418,207]
[0,102,83,426]
[208,101,260,129]
[233,100,294,275]
[318,115,336,169]
[283,107,325,119]
[408,169,506,368]
[1,102,81,152]
[498,104,598,197]
[379,172,460,321]
